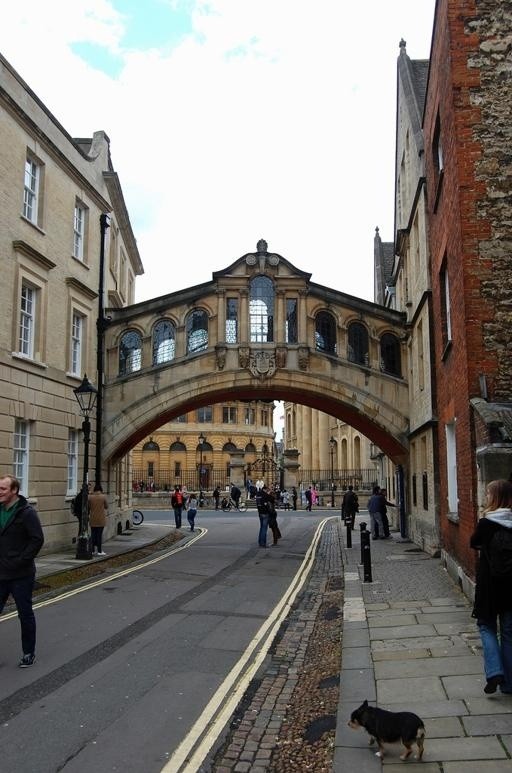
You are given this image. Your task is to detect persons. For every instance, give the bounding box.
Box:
[367,486,387,539]
[305,485,313,511]
[256,486,276,547]
[379,488,399,538]
[343,485,359,529]
[213,485,223,511]
[171,487,186,528]
[87,484,110,556]
[185,494,199,531]
[264,490,282,546]
[71,482,91,541]
[0,473,45,667]
[256,477,265,499]
[291,486,297,511]
[280,488,292,511]
[229,482,241,510]
[469,478,512,694]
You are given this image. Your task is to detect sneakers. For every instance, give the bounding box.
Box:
[97,552,106,556]
[18,652,38,670]
[92,550,97,556]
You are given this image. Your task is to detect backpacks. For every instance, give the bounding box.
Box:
[304,490,309,496]
[70,492,82,517]
[175,493,184,505]
[481,526,512,583]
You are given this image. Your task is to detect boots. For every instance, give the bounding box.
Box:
[277,526,282,541]
[270,525,277,547]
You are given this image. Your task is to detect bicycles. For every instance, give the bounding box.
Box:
[221,497,248,515]
[132,510,145,526]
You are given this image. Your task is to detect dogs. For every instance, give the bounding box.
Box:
[347,699,426,764]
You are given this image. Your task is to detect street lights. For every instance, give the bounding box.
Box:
[328,434,337,507]
[198,431,205,507]
[72,371,98,560]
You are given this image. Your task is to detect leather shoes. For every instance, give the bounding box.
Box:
[191,524,194,529]
[484,673,504,694]
